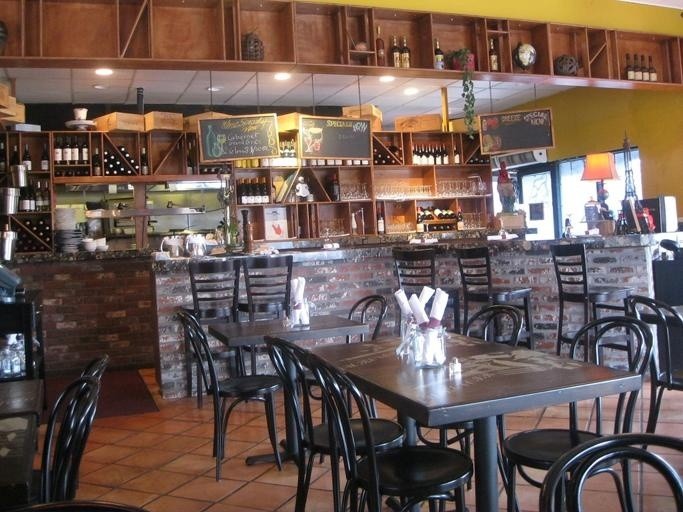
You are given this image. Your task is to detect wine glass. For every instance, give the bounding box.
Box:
[319,217,346,237]
[339,176,483,200]
[465,210,483,229]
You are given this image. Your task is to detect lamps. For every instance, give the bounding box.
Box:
[581,152,620,234]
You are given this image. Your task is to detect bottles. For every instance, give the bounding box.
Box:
[238,177,270,202]
[329,174,338,201]
[93,145,147,176]
[30,180,50,210]
[375,207,384,233]
[410,141,460,163]
[372,26,413,67]
[373,142,401,165]
[434,36,446,71]
[416,203,460,217]
[468,157,489,164]
[428,224,456,231]
[416,209,424,233]
[0,141,49,171]
[54,134,89,176]
[12,217,51,253]
[183,140,368,172]
[488,39,500,70]
[455,208,466,232]
[621,50,659,82]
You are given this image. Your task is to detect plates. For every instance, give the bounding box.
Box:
[53,208,82,252]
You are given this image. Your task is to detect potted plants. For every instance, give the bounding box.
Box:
[445,47,477,140]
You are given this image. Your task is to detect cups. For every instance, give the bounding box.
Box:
[289,300,314,331]
[402,318,448,367]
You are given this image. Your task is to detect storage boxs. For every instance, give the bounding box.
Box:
[449,115,481,132]
[89,112,144,131]
[342,104,383,130]
[395,114,442,131]
[495,215,524,230]
[184,112,232,132]
[278,112,303,130]
[144,110,183,132]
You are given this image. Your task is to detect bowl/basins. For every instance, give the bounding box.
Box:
[82,237,108,251]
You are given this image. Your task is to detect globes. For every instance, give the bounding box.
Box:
[513,41,537,74]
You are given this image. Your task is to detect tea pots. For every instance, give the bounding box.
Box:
[185,235,206,255]
[159,235,184,257]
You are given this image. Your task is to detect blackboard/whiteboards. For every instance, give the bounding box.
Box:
[478,108,555,155]
[197,113,280,163]
[299,115,374,160]
